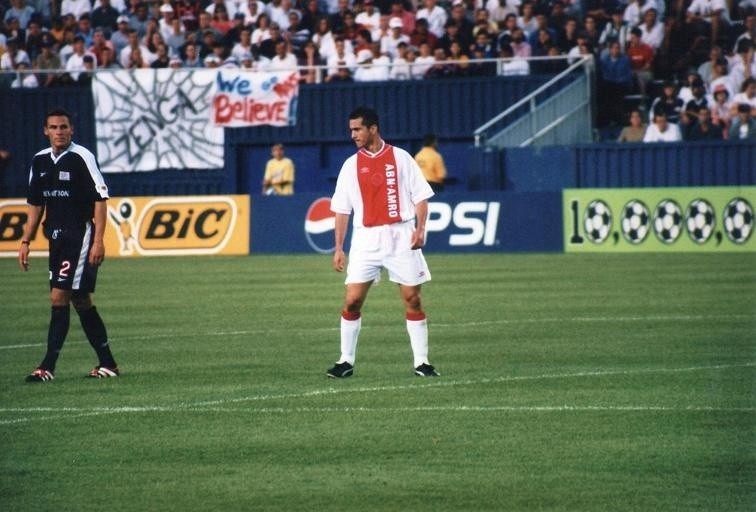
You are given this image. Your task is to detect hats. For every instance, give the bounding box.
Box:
[160,2,174,13]
[389,17,403,28]
[714,85,726,93]
[116,15,130,24]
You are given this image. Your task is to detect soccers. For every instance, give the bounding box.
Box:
[723,197,755,243]
[620,200,652,244]
[582,199,613,245]
[653,198,683,242]
[685,198,716,244]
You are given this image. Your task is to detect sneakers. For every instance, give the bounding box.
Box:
[415,363,440,377]
[86,366,119,378]
[26,368,54,382]
[327,362,353,378]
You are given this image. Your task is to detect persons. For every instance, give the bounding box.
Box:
[262,143,295,195]
[327,106,441,378]
[1,1,752,142]
[415,131,447,189]
[18,105,120,381]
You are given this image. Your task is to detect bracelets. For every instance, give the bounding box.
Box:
[22,241,30,246]
